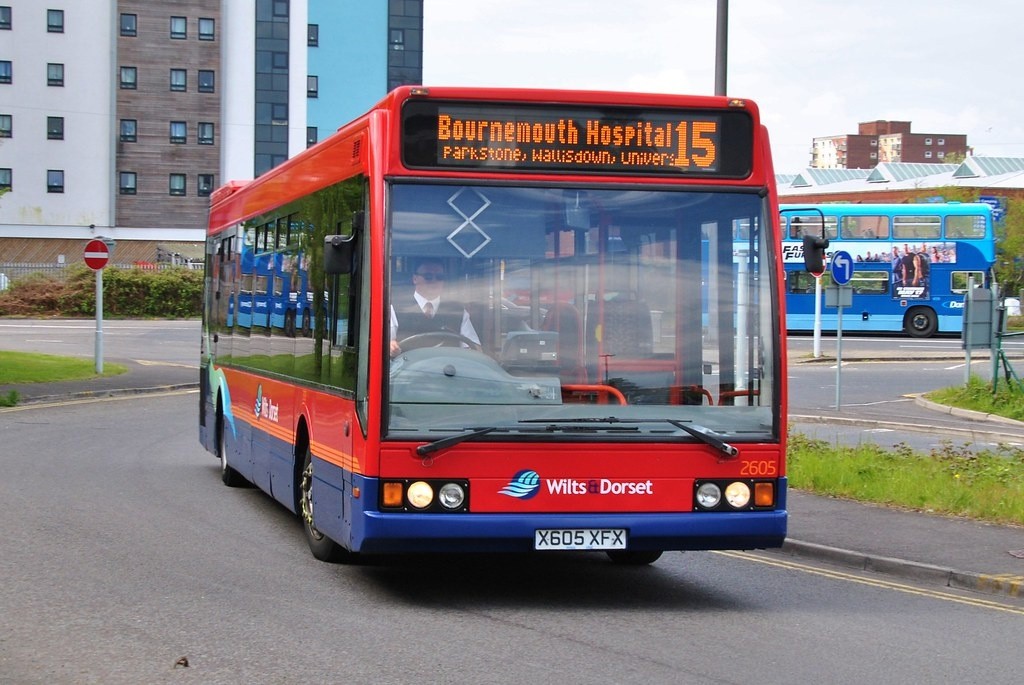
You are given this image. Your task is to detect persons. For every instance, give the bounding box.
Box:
[857,243,955,300]
[389,256,482,358]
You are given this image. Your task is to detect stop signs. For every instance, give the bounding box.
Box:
[83,240,109,272]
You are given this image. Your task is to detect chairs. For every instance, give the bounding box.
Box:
[780,223,973,297]
[386,291,677,404]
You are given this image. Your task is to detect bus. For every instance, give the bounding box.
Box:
[194,83,793,575]
[696,200,996,338]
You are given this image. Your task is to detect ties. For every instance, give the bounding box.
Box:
[424,302,433,320]
